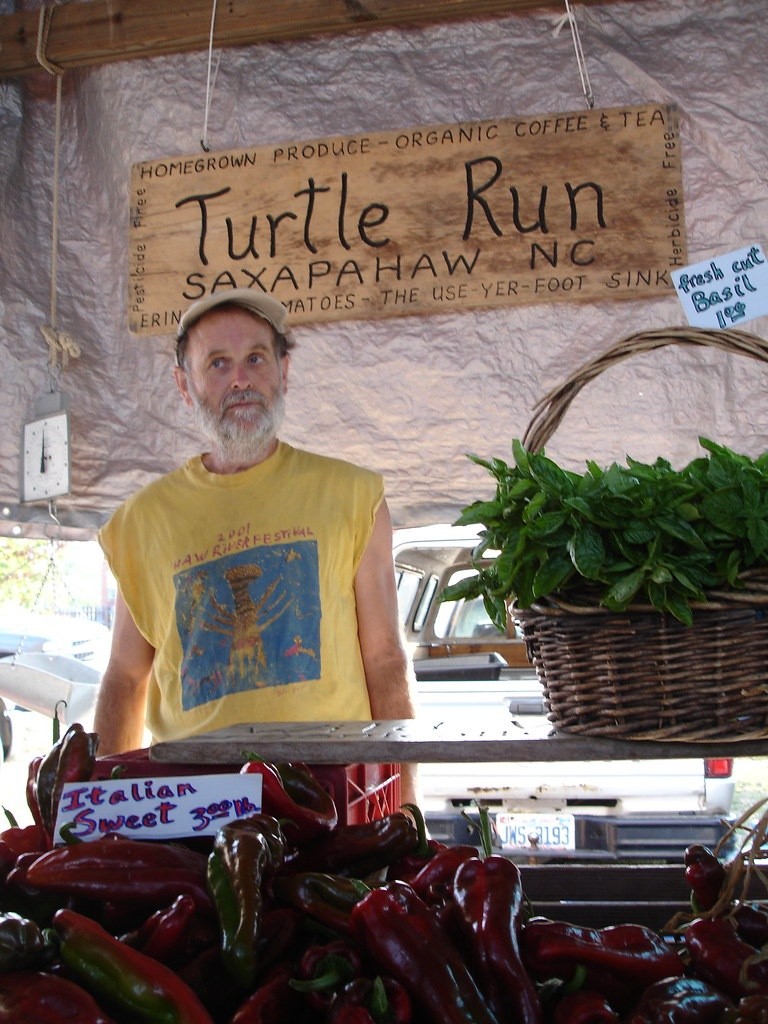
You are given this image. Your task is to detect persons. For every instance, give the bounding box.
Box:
[94,288,428,837]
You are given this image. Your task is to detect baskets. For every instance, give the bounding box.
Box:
[503,324,768,741]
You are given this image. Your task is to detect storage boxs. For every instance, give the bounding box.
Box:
[411,651,509,681]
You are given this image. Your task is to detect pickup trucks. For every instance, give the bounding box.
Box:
[387,523,736,863]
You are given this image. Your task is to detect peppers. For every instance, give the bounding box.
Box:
[0,722,767,1024]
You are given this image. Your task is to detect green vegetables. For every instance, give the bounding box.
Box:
[435,436,768,634]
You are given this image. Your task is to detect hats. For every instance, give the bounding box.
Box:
[174,290,288,366]
